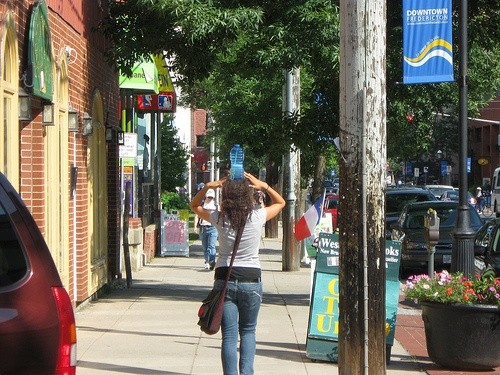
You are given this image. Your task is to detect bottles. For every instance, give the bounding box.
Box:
[230,144,245,180]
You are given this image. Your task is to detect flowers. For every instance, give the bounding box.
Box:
[400,269,500,305]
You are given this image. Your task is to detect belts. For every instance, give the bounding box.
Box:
[215,277,261,283]
[203,225,213,228]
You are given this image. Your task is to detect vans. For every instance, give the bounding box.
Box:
[491,168,500,217]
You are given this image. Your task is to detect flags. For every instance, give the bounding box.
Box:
[294,189,324,240]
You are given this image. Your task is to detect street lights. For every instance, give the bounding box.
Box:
[436,149,443,185]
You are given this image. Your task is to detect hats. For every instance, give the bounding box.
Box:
[205,189,215,198]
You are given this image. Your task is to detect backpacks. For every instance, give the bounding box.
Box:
[480,187,487,197]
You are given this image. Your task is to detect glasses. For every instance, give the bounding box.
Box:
[208,197,213,199]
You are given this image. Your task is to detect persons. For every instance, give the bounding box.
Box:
[476,180,492,211]
[189,171,286,375]
[197,189,220,270]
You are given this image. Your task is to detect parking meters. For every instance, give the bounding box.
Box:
[422,207,440,278]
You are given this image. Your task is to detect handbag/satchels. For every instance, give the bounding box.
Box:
[197,287,226,335]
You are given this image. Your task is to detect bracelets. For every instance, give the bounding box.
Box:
[265,185,269,192]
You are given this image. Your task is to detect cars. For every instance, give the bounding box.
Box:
[474,218,500,284]
[387,200,483,282]
[309,177,340,230]
[426,185,455,199]
[435,190,477,210]
[0,172,80,375]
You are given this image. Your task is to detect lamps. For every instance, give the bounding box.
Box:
[42,102,54,125]
[84,113,93,136]
[105,126,113,142]
[67,108,79,132]
[118,131,124,145]
[18,93,33,122]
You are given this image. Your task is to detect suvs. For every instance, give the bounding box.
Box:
[387,184,440,236]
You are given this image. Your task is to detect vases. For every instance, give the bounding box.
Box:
[417,301,500,369]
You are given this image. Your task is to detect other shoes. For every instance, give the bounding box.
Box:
[478,212,482,215]
[205,264,209,269]
[209,261,215,270]
